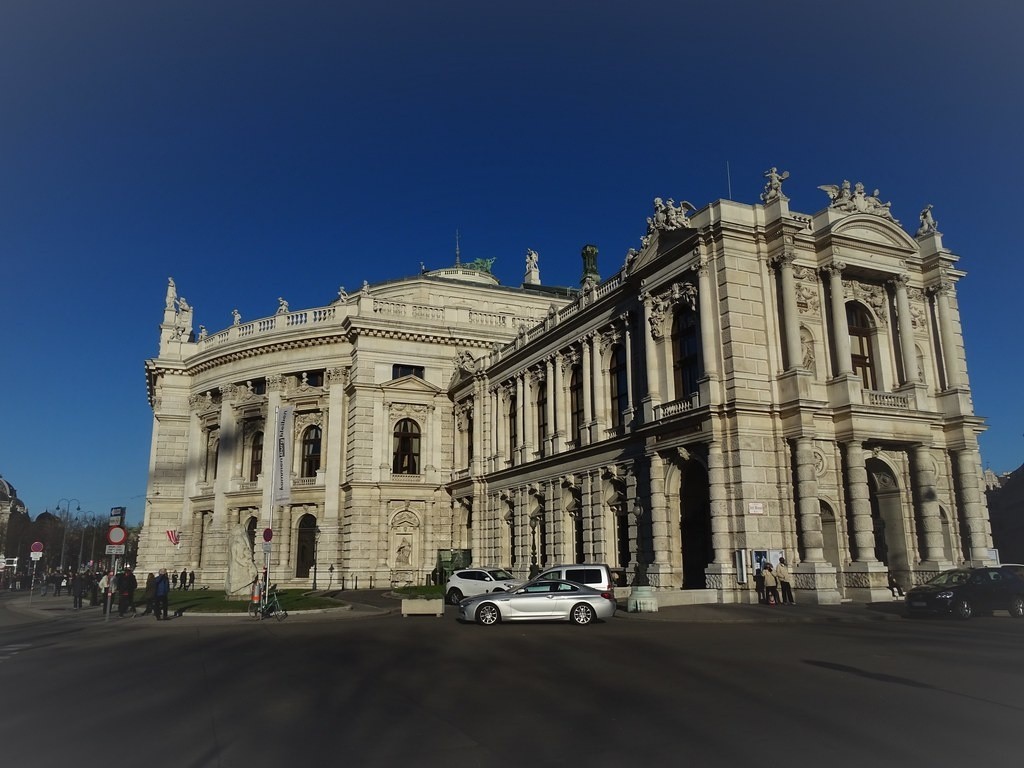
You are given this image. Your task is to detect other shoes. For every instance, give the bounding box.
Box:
[130,613,137,618]
[163,616,170,620]
[892,595,896,597]
[899,594,905,597]
[156,615,163,620]
[791,602,796,605]
[74,608,77,610]
[119,614,125,618]
[783,602,789,605]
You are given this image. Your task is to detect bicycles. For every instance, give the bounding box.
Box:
[248,592,284,620]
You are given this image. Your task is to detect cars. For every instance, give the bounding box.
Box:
[976,564,1024,617]
[445,567,523,604]
[904,567,985,620]
[459,579,617,627]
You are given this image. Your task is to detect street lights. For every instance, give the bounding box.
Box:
[55,498,80,574]
[74,511,95,571]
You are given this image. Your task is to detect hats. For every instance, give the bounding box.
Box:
[159,567,168,574]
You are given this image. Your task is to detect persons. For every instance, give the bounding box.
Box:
[178,568,187,590]
[232,310,242,324]
[144,569,170,621]
[762,556,796,607]
[640,197,691,247]
[116,569,137,617]
[526,249,538,270]
[363,281,368,291]
[172,570,178,589]
[198,325,206,340]
[887,568,905,597]
[166,276,176,308]
[338,286,348,301]
[841,179,901,226]
[9,564,115,613]
[277,298,289,313]
[186,571,194,590]
[920,204,934,233]
[175,297,190,313]
[763,168,782,202]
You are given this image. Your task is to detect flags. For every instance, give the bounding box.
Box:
[168,530,178,545]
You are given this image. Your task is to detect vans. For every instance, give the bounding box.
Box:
[530,564,614,593]
